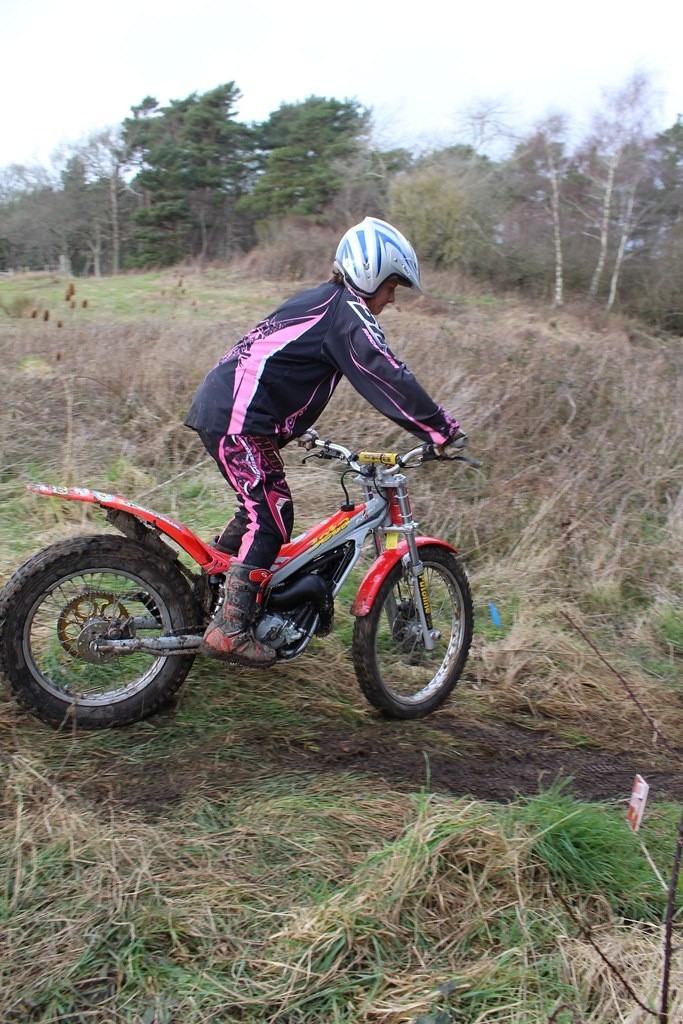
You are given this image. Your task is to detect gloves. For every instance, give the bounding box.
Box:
[294,428,319,451]
[431,430,467,460]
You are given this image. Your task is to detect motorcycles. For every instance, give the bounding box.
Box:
[0,430,493,732]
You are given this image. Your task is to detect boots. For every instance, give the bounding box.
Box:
[208,535,238,560]
[199,563,278,669]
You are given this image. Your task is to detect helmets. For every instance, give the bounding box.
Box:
[334,217,423,298]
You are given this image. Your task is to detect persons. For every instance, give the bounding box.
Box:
[184,216,469,669]
